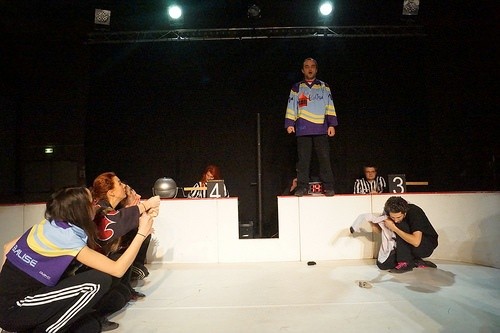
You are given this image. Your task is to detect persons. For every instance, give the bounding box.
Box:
[283,162,320,195]
[286,57,338,196]
[370,196,439,274]
[0,185,154,333]
[59,172,160,331]
[188,166,229,198]
[353,162,386,194]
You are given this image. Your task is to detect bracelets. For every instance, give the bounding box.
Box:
[142,203,146,211]
[137,233,146,238]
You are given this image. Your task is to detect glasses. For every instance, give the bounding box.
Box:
[89,197,97,207]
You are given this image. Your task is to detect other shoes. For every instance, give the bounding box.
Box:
[130,291,146,301]
[295,187,308,196]
[101,318,119,330]
[325,189,335,196]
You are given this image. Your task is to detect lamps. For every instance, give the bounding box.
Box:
[42,143,57,194]
[247,4,262,21]
[95,8,111,29]
[402,0,420,16]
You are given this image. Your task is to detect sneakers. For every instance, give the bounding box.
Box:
[413,257,437,268]
[391,262,413,273]
